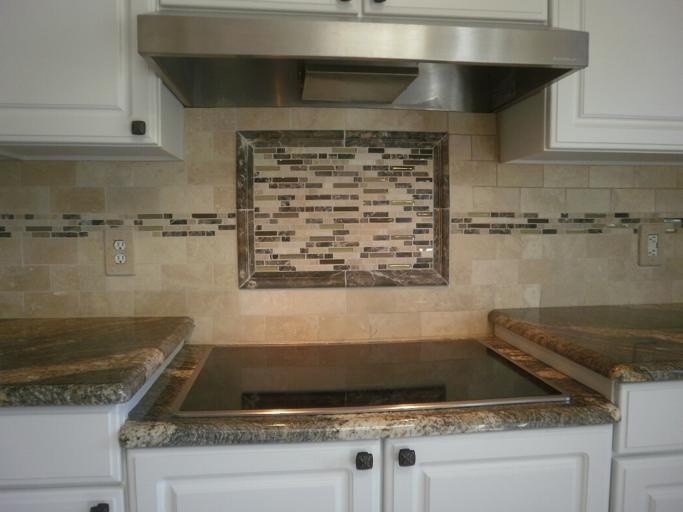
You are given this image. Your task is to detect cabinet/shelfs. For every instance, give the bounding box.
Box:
[487,321,683,512]
[0,336,186,512]
[0,0,187,162]
[122,422,614,512]
[498,0,682,166]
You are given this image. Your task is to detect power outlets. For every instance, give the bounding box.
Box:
[639,224,665,267]
[104,227,134,277]
